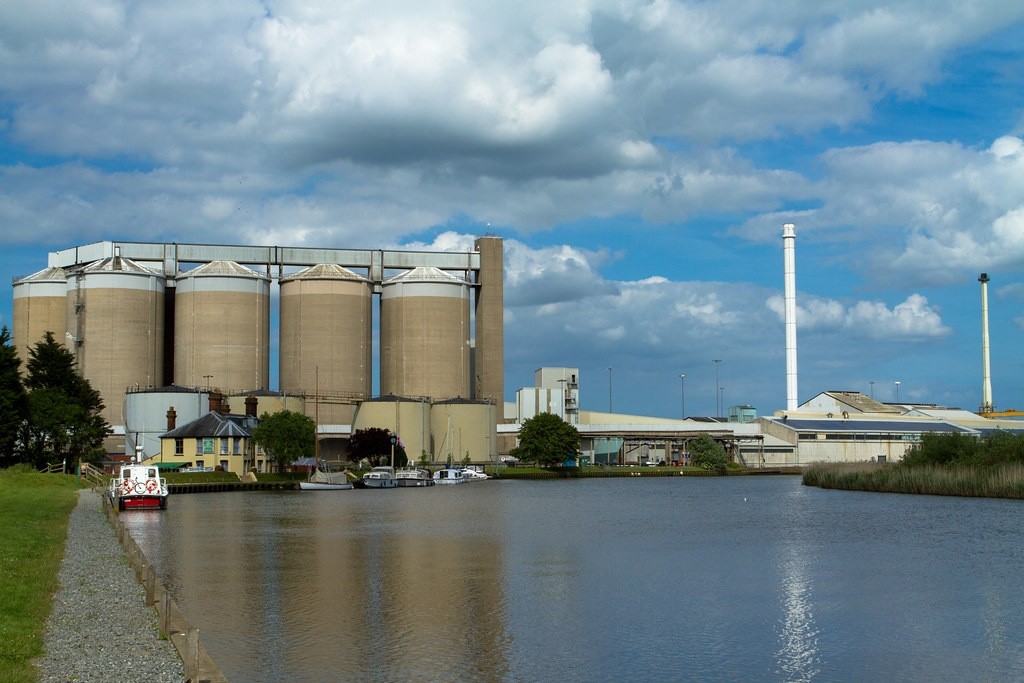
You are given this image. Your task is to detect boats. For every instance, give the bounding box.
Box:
[394,459,430,487]
[362,466,397,488]
[110,446,169,512]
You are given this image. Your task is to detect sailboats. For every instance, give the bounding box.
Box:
[299,365,355,491]
[432,415,488,485]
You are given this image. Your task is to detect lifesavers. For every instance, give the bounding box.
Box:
[124,479,135,492]
[146,479,157,492]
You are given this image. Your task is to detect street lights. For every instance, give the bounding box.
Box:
[869,381,874,400]
[680,374,687,420]
[720,387,725,418]
[606,366,615,413]
[895,381,900,404]
[711,358,722,419]
